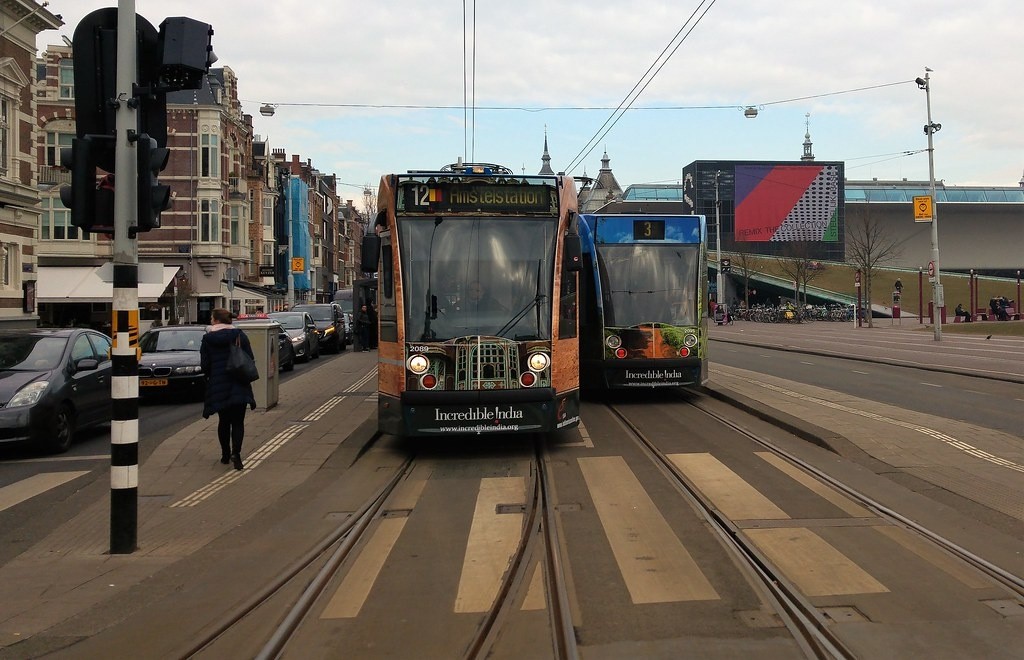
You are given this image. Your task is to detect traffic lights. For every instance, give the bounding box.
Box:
[56,135,96,227]
[136,135,176,230]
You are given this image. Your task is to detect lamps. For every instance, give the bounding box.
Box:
[260,104,274,116]
[176,274,187,282]
[364,189,371,195]
[744,106,758,118]
[616,193,623,204]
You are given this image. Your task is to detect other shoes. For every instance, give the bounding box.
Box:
[230,452,244,470]
[221,455,229,464]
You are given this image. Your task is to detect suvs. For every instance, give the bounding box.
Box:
[289,302,352,351]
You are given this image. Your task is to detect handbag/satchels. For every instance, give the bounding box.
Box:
[227,332,259,384]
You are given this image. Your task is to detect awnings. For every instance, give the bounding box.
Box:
[36,266,180,302]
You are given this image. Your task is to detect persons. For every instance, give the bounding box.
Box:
[453,280,487,319]
[895,277,903,293]
[708,298,716,318]
[955,303,972,322]
[990,296,1015,321]
[356,304,371,352]
[200,308,257,471]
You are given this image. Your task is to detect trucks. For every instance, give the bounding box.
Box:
[331,289,354,342]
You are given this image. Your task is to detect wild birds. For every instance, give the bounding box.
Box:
[884,307,888,310]
[984,334,992,341]
[924,324,931,328]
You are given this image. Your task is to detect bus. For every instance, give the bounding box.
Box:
[361,159,596,437]
[575,199,713,388]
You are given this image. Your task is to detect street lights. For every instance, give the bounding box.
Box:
[915,67,945,339]
[715,168,725,307]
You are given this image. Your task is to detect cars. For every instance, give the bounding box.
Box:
[269,319,295,373]
[0,328,112,456]
[132,325,208,403]
[266,312,322,363]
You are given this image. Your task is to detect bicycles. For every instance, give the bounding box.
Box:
[725,300,861,323]
